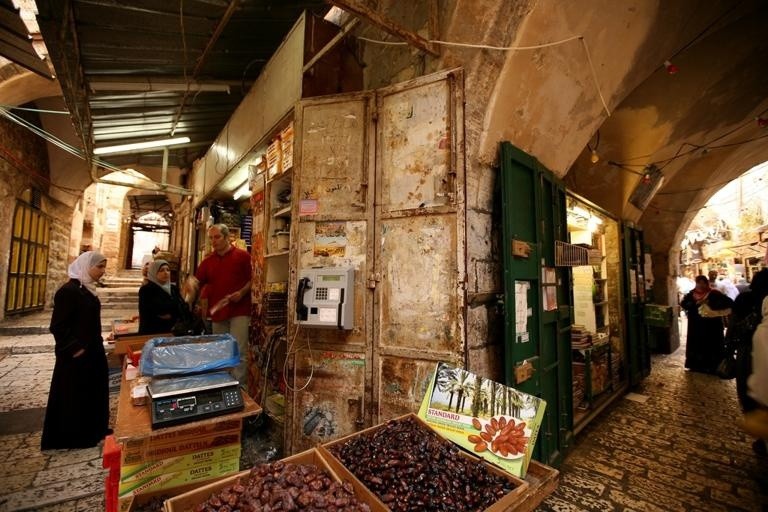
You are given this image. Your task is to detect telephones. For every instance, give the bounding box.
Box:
[295,264,353,332]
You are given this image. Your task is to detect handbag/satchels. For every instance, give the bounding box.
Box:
[719,358,738,379]
[698,290,734,318]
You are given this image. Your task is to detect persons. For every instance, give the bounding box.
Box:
[41,250,113,452]
[680,266,767,472]
[189,223,253,392]
[137,260,189,335]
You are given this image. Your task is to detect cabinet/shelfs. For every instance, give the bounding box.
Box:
[261,173,292,258]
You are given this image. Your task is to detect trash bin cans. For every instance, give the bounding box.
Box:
[646,304,680,354]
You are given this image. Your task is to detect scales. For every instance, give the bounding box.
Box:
[145,372,244,432]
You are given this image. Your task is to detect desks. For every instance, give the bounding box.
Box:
[110,331,261,511]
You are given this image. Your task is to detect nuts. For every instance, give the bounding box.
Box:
[331,415,519,512]
[468,416,528,456]
[193,461,372,512]
[131,493,172,512]
